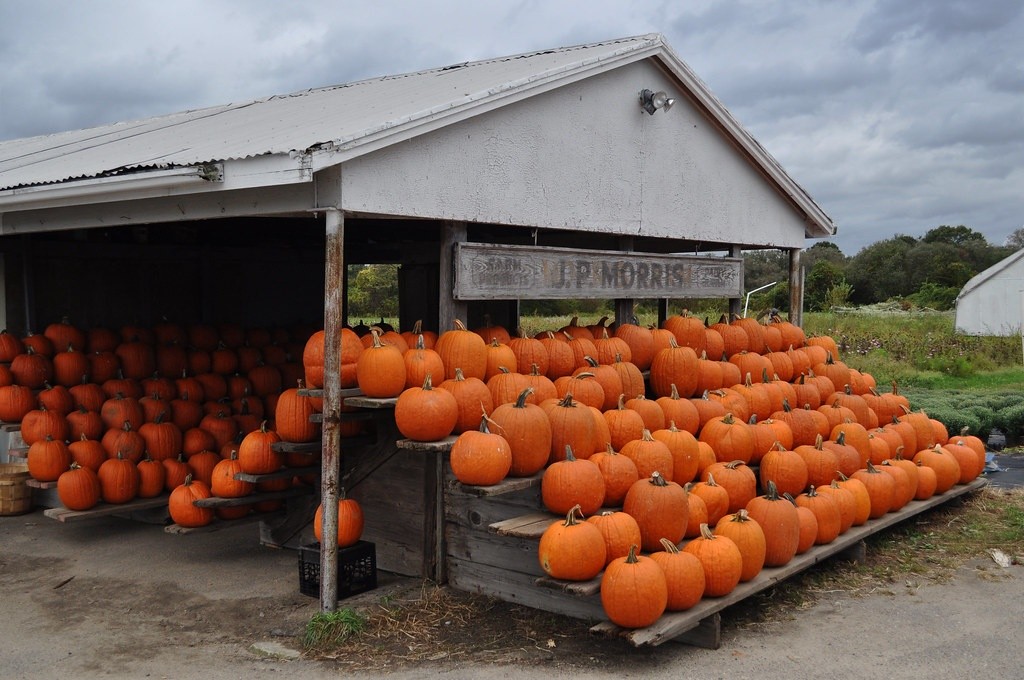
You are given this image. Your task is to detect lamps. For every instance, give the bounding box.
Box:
[642,89,678,116]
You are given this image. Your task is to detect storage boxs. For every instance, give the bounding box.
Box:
[298,540,378,601]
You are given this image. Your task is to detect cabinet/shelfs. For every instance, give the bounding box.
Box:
[0,325,987,650]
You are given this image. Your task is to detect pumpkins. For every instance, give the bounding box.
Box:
[0,317,395,549]
[303,309,986,628]
